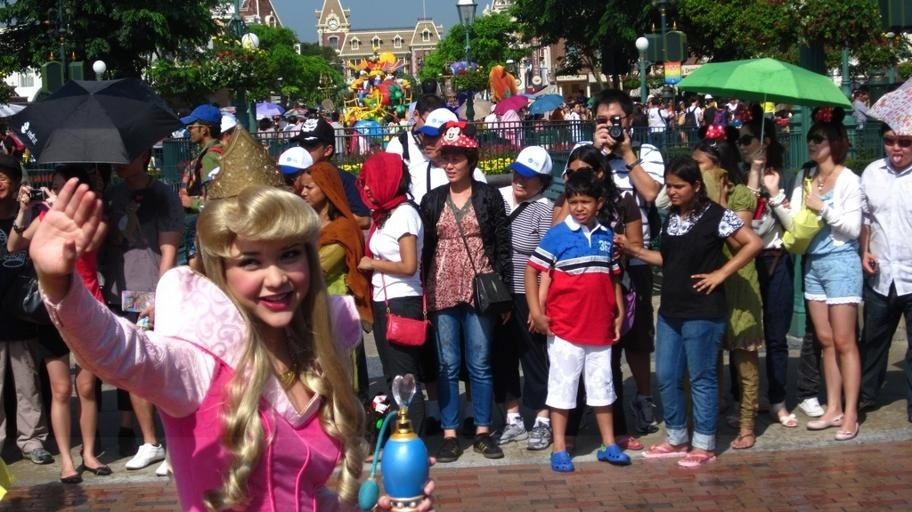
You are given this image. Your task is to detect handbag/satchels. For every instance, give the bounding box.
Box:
[474,273,512,313]
[6,269,51,324]
[386,314,431,347]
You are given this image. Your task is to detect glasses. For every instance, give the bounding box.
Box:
[807,134,828,144]
[736,134,758,146]
[186,125,204,130]
[883,137,912,147]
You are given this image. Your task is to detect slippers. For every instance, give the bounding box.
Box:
[726,415,755,448]
[597,435,716,467]
[550,451,573,471]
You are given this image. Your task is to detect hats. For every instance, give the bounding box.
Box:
[510,146,553,177]
[414,107,478,152]
[180,104,237,133]
[277,118,335,174]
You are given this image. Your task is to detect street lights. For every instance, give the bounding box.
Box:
[240,31,261,133]
[634,36,650,104]
[92,59,107,80]
[456,0,477,120]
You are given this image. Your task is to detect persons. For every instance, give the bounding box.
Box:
[29,124,437,510]
[4,102,242,489]
[272,74,912,472]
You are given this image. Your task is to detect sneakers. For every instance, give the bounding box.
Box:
[630,392,658,433]
[22,448,52,464]
[125,443,168,476]
[473,421,552,458]
[436,437,462,462]
[777,397,859,440]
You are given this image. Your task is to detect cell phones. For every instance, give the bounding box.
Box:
[30,190,46,201]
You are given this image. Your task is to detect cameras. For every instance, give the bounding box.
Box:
[608,115,625,143]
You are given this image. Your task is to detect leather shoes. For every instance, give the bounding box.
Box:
[60,459,111,483]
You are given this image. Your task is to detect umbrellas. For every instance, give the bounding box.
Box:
[674,55,856,147]
